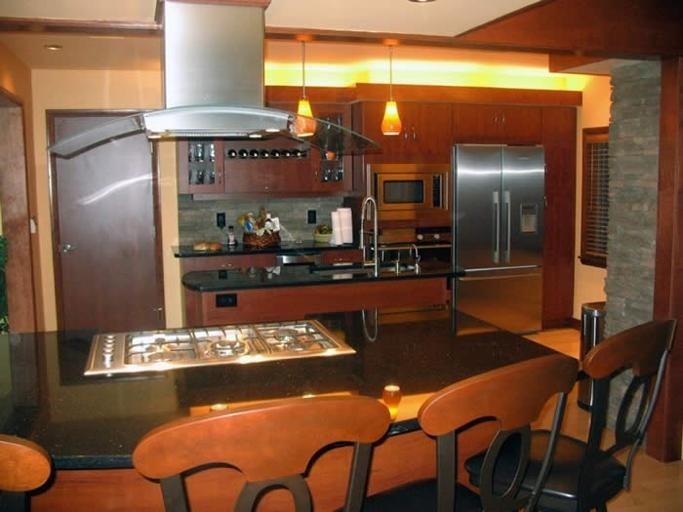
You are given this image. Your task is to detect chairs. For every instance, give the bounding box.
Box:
[132,396,390,512]
[336,354,579,512]
[0,434,54,512]
[464,318,678,512]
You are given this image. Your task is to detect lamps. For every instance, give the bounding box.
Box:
[381,39,402,135]
[295,35,317,137]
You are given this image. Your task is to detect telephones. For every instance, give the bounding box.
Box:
[227,225,237,249]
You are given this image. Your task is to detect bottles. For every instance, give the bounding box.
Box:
[226,226,238,246]
[227,148,302,159]
[186,143,216,184]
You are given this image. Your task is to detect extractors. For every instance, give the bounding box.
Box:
[47,4,381,164]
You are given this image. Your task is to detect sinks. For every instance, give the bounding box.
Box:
[381,263,415,273]
[313,267,376,277]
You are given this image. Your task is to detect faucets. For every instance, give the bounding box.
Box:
[407,244,422,275]
[358,196,380,277]
[361,305,378,342]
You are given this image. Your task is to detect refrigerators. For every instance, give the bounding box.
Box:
[452,145,545,335]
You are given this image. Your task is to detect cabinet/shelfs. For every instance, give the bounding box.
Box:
[178,139,224,195]
[541,105,578,331]
[320,249,363,264]
[186,254,277,272]
[311,104,354,193]
[453,102,542,146]
[352,100,453,164]
[223,137,312,193]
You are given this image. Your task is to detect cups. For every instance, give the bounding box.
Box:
[325,152,335,160]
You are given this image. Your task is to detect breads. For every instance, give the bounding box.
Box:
[193,242,223,250]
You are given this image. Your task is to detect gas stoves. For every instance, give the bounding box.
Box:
[81,317,359,376]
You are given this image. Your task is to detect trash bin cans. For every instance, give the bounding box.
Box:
[577,302,606,412]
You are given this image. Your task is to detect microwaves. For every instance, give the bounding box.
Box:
[376,174,443,210]
